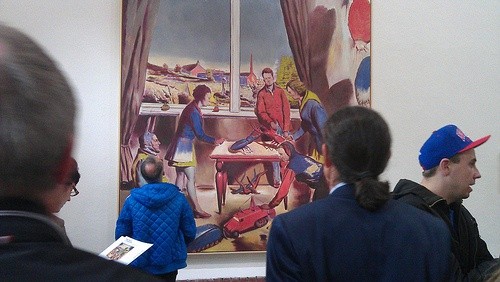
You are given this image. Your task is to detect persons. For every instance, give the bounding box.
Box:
[390,124,500,282]
[115,154,197,282]
[49,158,80,231]
[0,23,161,282]
[266,105,452,282]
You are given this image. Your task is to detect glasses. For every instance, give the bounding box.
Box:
[65,182,79,197]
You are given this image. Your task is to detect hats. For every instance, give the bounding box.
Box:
[60,156,80,182]
[418,124,491,170]
